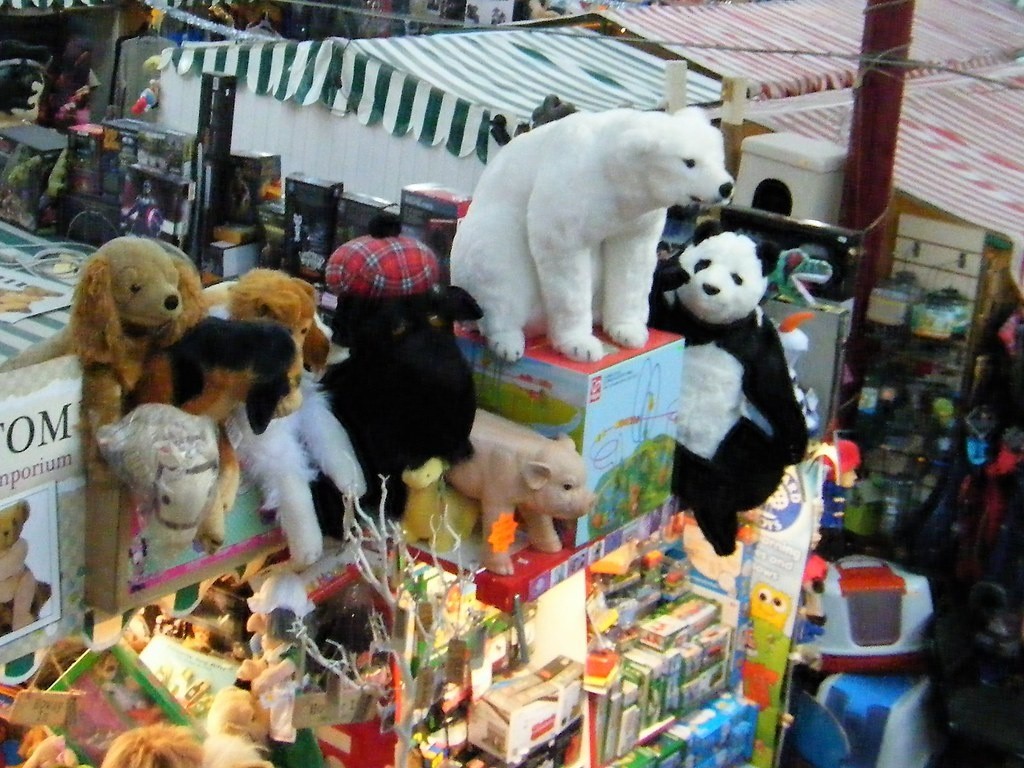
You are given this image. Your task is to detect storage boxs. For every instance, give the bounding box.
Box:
[0,116,1014,768]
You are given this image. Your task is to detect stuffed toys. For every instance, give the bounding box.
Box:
[450,107,736,364]
[101,208,484,571]
[0,499,36,632]
[648,220,808,557]
[20,649,276,768]
[0,235,207,485]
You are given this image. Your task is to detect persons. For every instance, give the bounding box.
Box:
[234,567,324,768]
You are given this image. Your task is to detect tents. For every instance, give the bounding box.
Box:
[154,1,1024,243]
